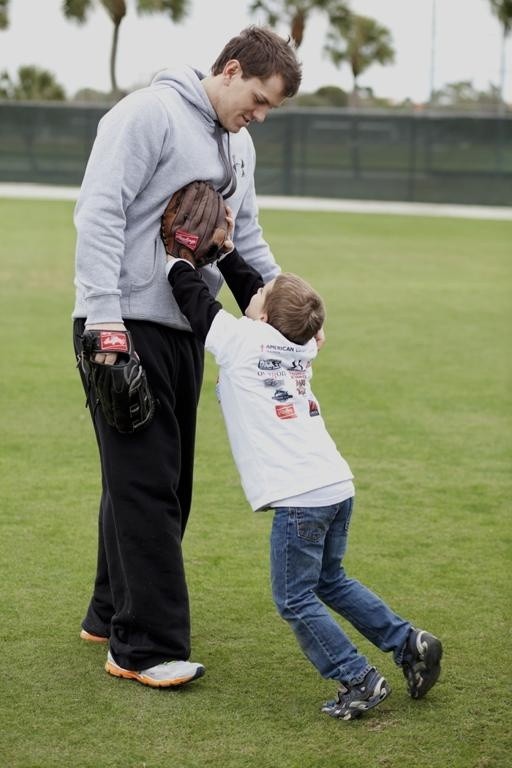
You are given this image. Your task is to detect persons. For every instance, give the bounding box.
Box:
[71,24,328,687]
[159,180,442,721]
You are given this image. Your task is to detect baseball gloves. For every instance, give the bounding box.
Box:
[160,180,229,267]
[74,330,154,435]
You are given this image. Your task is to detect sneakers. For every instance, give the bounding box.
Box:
[104,649,206,688]
[320,665,392,721]
[402,626,442,700]
[79,628,109,642]
